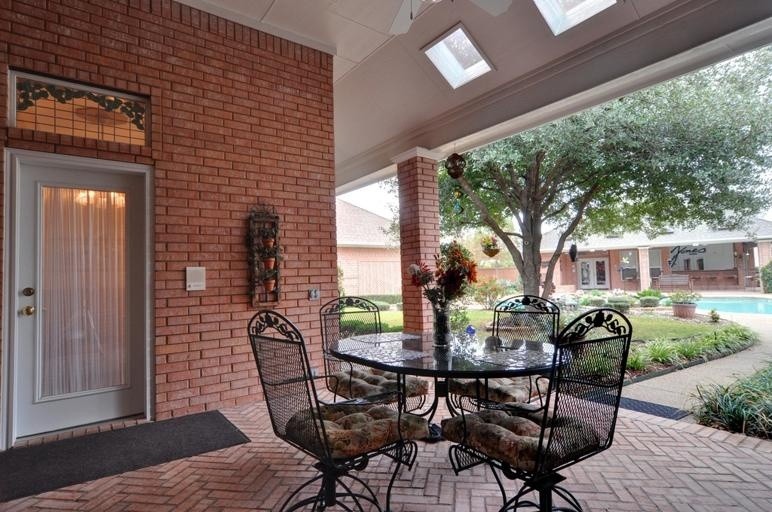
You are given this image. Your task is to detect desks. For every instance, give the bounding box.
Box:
[329,332,572,458]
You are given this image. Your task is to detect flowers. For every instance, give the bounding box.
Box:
[410,240,478,306]
[479,237,498,249]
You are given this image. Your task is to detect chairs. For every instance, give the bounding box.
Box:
[445,294,560,418]
[319,295,429,413]
[440,307,633,512]
[247,309,429,512]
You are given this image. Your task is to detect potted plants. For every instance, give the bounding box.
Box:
[250,207,280,292]
[669,290,702,320]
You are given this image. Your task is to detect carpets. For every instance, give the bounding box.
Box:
[587,393,694,420]
[0,408,253,503]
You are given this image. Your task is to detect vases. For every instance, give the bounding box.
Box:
[432,306,450,347]
[482,249,501,257]
